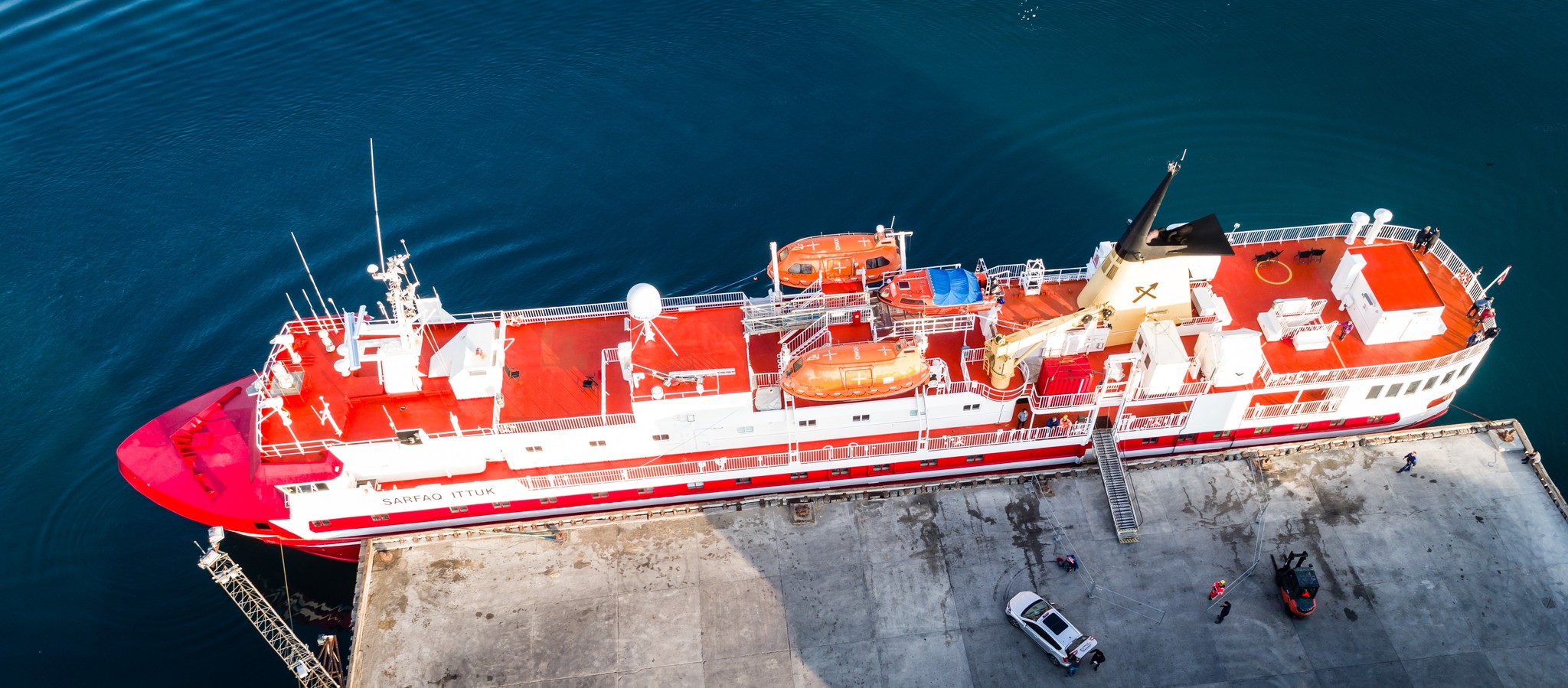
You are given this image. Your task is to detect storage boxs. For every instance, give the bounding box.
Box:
[1037,353,1093,405]
[1190,287,1216,317]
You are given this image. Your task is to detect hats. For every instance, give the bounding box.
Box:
[1524,451,1529,454]
[1426,226,1431,230]
[1478,330,1483,333]
[1435,228,1440,232]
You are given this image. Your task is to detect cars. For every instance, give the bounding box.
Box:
[1006,591,1097,667]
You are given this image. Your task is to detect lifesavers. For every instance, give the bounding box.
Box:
[507,315,522,326]
[1459,271,1469,285]
[1150,417,1163,426]
[953,437,967,446]
[1280,374,1293,385]
[499,424,515,433]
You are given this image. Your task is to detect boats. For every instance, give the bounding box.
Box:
[779,340,930,402]
[112,133,1499,565]
[878,264,997,313]
[767,230,901,293]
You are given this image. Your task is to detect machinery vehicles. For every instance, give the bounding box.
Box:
[1270,551,1320,620]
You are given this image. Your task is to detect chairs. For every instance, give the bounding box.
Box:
[1252,254,1270,269]
[581,371,599,394]
[1266,248,1285,266]
[1310,246,1326,263]
[1293,249,1313,266]
[504,363,523,385]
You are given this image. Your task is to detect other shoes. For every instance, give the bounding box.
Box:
[1415,248,1418,251]
[1521,459,1527,461]
[1341,338,1343,342]
[1466,314,1471,317]
[1475,321,1477,324]
[1208,595,1212,600]
[1396,471,1400,473]
[1522,462,1528,464]
[1404,468,1410,471]
[1472,317,1477,320]
[1218,615,1220,619]
[1412,247,1415,250]
[1421,252,1425,256]
[1475,325,1480,328]
[1337,336,1342,338]
[1215,621,1221,623]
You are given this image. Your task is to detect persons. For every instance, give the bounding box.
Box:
[1337,320,1354,342]
[1046,416,1058,432]
[1017,408,1028,429]
[1483,327,1501,342]
[1421,227,1440,256]
[1466,330,1484,348]
[1215,601,1231,623]
[1412,226,1431,251]
[1475,308,1496,328]
[1522,450,1541,465]
[1465,297,1494,320]
[1208,580,1225,600]
[1396,452,1417,473]
[1059,414,1072,435]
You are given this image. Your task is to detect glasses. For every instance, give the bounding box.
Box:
[1220,582,1222,585]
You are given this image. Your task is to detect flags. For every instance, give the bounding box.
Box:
[1498,272,1508,285]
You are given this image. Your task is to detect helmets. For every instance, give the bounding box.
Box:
[1220,580,1225,585]
